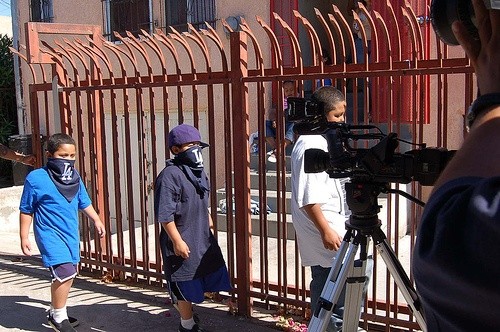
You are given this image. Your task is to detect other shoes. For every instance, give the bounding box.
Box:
[267,149,277,155]
[193,313,200,323]
[268,153,278,163]
[178,321,205,332]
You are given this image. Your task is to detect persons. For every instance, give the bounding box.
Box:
[291,85,372,332]
[0,144,36,166]
[19,133,106,332]
[353,0,371,87]
[413,0,500,332]
[266,82,300,163]
[155,125,233,332]
[315,48,333,87]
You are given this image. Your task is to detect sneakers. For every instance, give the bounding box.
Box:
[48,312,79,327]
[47,314,78,332]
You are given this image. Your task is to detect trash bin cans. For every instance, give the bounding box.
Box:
[8,133,47,186]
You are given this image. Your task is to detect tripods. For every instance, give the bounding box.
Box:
[305,181,427,331]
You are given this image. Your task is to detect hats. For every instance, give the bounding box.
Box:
[165,124,210,149]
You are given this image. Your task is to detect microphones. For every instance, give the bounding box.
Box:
[291,121,375,135]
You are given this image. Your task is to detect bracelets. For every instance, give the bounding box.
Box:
[20,155,26,163]
[466,92,500,128]
